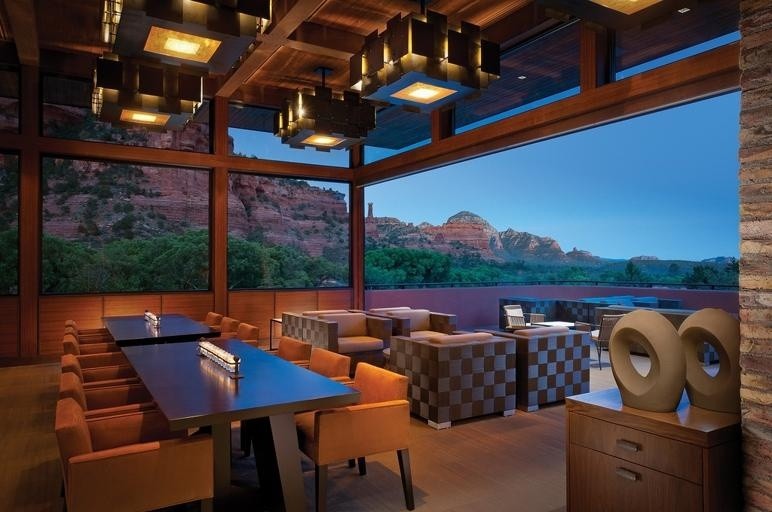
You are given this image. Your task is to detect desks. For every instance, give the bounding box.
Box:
[103,313,362,512]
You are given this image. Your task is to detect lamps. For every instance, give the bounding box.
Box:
[348,0,503,116]
[273,67,377,152]
[91,0,271,131]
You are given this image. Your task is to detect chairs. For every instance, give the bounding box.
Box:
[200,310,416,512]
[53,320,216,512]
[498,295,739,371]
[347,305,592,431]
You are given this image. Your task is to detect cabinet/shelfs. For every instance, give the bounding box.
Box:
[564,387,744,512]
